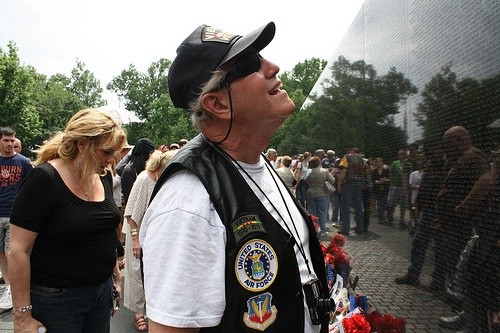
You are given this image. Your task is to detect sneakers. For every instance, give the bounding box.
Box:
[0,284,13,309]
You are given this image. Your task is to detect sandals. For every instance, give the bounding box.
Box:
[133,313,147,331]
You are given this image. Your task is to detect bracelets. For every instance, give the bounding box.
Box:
[11,305,32,314]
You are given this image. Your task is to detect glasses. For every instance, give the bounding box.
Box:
[220,49,263,89]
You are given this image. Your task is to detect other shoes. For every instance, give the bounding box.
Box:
[337,228,349,233]
[399,222,409,227]
[439,312,476,331]
[320,232,328,236]
[332,221,337,227]
[351,226,368,233]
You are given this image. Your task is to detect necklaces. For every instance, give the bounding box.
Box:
[65,161,88,196]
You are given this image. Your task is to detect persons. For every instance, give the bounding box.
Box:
[0,128,34,308]
[393,119,500,333]
[138,22,334,333]
[8,109,126,333]
[292,144,425,235]
[105,138,189,331]
[264,148,294,194]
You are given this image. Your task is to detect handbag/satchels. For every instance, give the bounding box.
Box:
[324,181,336,193]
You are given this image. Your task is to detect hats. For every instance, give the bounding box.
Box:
[168,20,275,106]
[314,149,324,155]
[263,149,277,156]
[170,142,179,148]
[445,125,468,140]
[132,138,154,155]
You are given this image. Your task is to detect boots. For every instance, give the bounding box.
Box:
[431,273,458,300]
[395,269,421,284]
